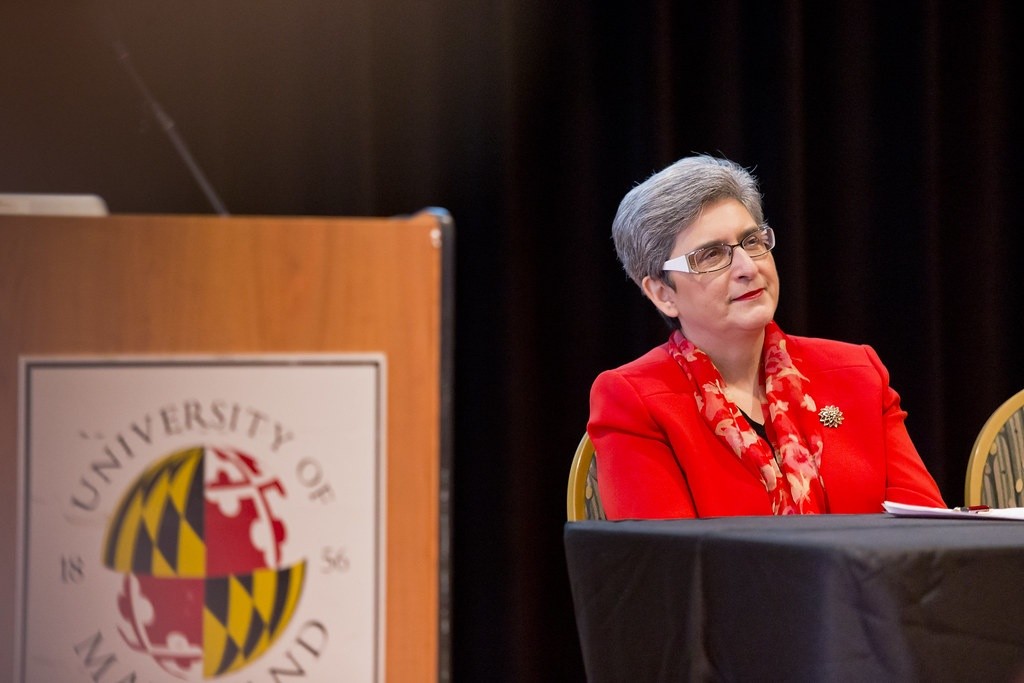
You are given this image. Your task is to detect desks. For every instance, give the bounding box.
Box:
[564,512,1024,683]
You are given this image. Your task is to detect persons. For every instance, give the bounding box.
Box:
[586,155,948,521]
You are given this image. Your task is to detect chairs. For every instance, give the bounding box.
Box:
[965,391,1024,511]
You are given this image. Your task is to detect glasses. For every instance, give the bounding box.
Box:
[662,225,776,274]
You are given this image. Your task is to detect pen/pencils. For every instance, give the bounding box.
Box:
[967,505,990,513]
[954,506,968,512]
